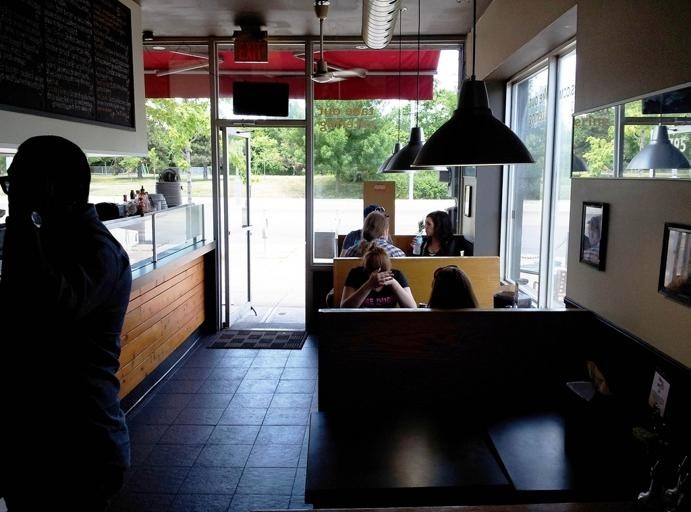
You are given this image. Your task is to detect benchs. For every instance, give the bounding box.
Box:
[317,305,593,410]
[332,256,501,307]
[335,233,464,255]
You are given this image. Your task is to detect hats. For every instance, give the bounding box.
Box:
[364,205,390,218]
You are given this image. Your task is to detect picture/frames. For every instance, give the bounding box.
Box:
[655,222,691,307]
[578,200,610,273]
[464,184,472,217]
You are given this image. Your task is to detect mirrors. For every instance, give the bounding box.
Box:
[570,81,691,182]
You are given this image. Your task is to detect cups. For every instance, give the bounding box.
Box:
[155,201,161,211]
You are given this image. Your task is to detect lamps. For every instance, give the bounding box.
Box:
[625,115,690,171]
[383,0,449,172]
[411,0,535,167]
[375,0,406,175]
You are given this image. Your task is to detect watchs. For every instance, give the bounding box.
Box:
[6,209,43,231]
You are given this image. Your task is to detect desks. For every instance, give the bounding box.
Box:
[304,412,654,509]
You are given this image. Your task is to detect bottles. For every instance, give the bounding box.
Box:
[115,187,151,216]
[412,231,423,255]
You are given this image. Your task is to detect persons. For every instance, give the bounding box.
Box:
[425,264,480,309]
[338,245,417,308]
[409,210,472,256]
[584,216,602,265]
[343,211,408,258]
[1,134,134,512]
[338,204,394,257]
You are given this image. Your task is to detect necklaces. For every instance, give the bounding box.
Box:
[430,242,440,252]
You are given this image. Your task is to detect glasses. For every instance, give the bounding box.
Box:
[0,175,19,195]
[375,207,385,213]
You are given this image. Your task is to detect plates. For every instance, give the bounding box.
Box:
[149,193,168,211]
[156,180,183,206]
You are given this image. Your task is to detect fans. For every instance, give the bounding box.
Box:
[265,0,369,84]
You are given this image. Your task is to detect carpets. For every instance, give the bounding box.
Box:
[206,330,309,349]
